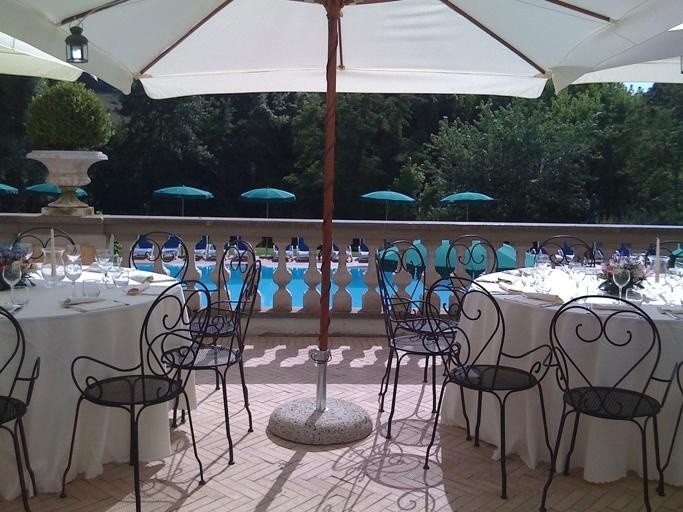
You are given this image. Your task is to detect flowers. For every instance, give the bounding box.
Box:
[0,239,43,290]
[596,250,651,295]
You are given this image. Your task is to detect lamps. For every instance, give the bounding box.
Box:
[64,17,89,64]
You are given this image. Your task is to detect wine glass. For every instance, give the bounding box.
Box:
[14,242,34,259]
[99,249,114,291]
[3,264,22,309]
[664,269,681,306]
[535,255,550,267]
[612,269,630,305]
[63,245,83,299]
[570,267,587,297]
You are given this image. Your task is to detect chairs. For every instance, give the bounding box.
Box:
[379,239,461,413]
[273,245,293,263]
[296,237,309,262]
[133,235,155,260]
[358,237,378,262]
[375,247,471,441]
[189,241,256,390]
[557,242,574,267]
[161,236,183,260]
[332,242,352,263]
[446,234,499,306]
[537,240,561,262]
[208,240,231,261]
[167,260,262,465]
[11,226,77,260]
[128,231,189,285]
[613,242,630,263]
[645,240,683,269]
[195,235,209,262]
[425,277,557,499]
[540,295,678,512]
[0,305,40,512]
[234,236,248,261]
[60,279,212,512]
[534,235,595,268]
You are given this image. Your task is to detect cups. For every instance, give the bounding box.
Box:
[12,286,31,307]
[625,287,642,307]
[82,278,100,297]
[522,271,534,286]
[113,270,131,288]
